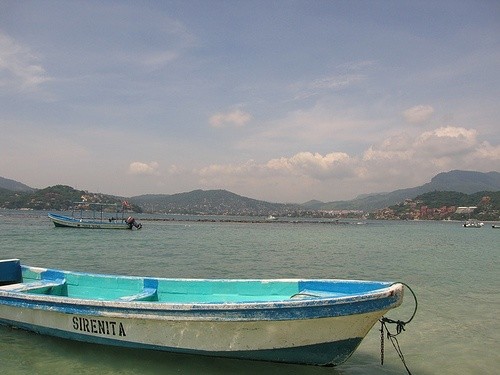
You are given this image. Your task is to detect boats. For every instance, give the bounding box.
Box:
[48,202,142,230]
[463,221,483,228]
[0,259,403,368]
[265,216,278,220]
